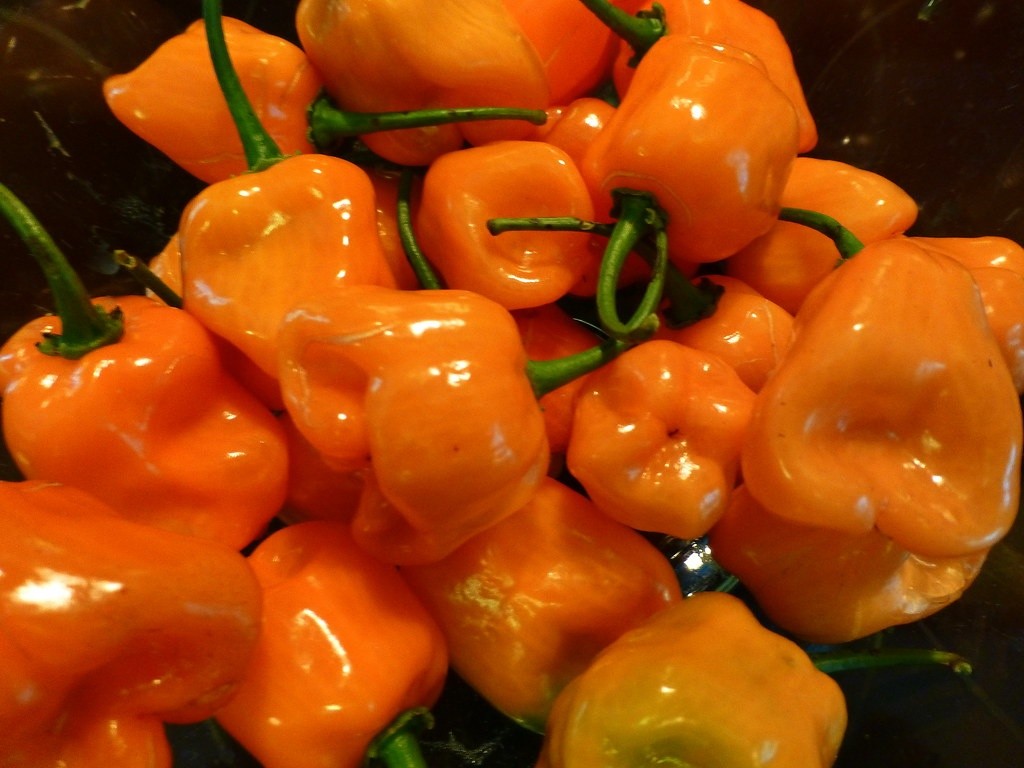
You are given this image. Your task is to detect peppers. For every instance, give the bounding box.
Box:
[0,0,1024,768]
